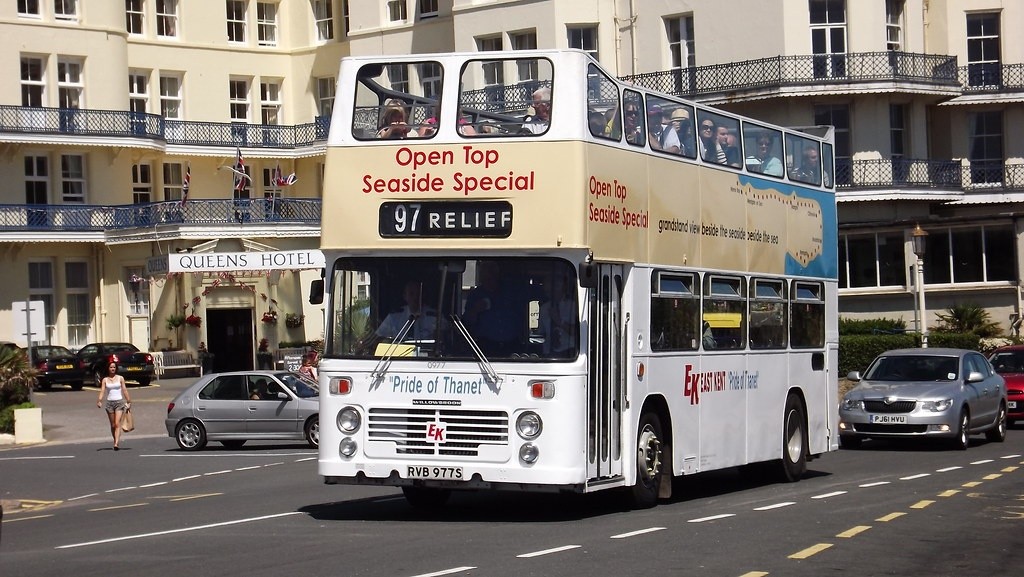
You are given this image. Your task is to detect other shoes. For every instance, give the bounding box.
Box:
[114,445,118,450]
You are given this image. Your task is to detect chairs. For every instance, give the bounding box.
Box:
[268,382,278,395]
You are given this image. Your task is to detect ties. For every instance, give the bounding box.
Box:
[407,314,415,340]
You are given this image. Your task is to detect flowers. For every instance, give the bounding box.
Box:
[286,312,306,327]
[185,315,201,327]
[262,305,277,325]
[197,341,208,353]
[259,338,269,352]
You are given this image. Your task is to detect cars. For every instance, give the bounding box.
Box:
[988,345,1024,429]
[74,342,155,388]
[164,371,319,451]
[22,347,85,391]
[838,348,1008,450]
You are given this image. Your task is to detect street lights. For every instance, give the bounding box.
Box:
[909,224,928,346]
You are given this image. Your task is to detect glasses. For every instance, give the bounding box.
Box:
[700,125,715,132]
[626,110,639,117]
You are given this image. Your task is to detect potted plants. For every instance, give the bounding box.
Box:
[166,315,185,327]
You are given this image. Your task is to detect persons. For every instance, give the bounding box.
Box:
[590,102,643,145]
[703,311,715,349]
[648,103,692,153]
[459,119,476,136]
[756,131,784,177]
[462,261,577,359]
[699,119,742,168]
[97,361,132,449]
[250,379,268,400]
[375,277,449,358]
[300,351,319,380]
[375,98,438,137]
[789,146,830,188]
[521,89,551,133]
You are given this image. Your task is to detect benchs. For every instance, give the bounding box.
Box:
[149,351,204,381]
[273,346,310,369]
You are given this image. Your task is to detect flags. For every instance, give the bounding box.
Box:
[182,165,191,204]
[271,164,299,186]
[237,150,247,191]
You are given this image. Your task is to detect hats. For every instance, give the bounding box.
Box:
[647,104,662,116]
[669,109,689,122]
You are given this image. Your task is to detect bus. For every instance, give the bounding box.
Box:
[309,49,841,508]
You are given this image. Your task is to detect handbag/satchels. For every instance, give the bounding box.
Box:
[121,403,134,432]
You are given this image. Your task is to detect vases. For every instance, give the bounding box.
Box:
[198,352,212,375]
[257,351,272,370]
